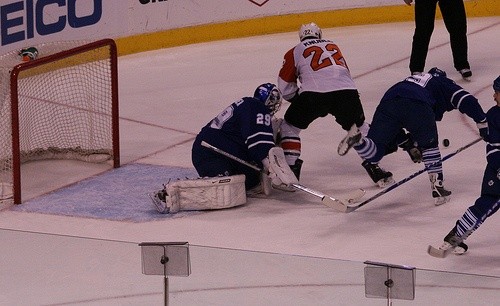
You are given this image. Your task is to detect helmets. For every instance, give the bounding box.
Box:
[429,67,446,76]
[300,23,321,41]
[254,83,282,111]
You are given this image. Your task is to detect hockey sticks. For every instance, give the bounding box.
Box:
[200,140,366,206]
[321,136,484,214]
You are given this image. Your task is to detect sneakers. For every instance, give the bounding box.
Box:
[459,68,473,81]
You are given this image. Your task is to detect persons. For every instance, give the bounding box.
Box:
[441,76,500,254]
[150,83,282,213]
[403,0,474,82]
[336,66,488,206]
[269,23,397,191]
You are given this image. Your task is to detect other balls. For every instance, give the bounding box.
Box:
[443,139,450,147]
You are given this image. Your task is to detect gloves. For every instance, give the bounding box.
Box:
[399,133,423,164]
[479,122,490,142]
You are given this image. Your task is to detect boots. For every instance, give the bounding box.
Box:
[439,220,468,254]
[270,159,303,192]
[337,124,366,156]
[428,172,453,205]
[362,160,396,188]
